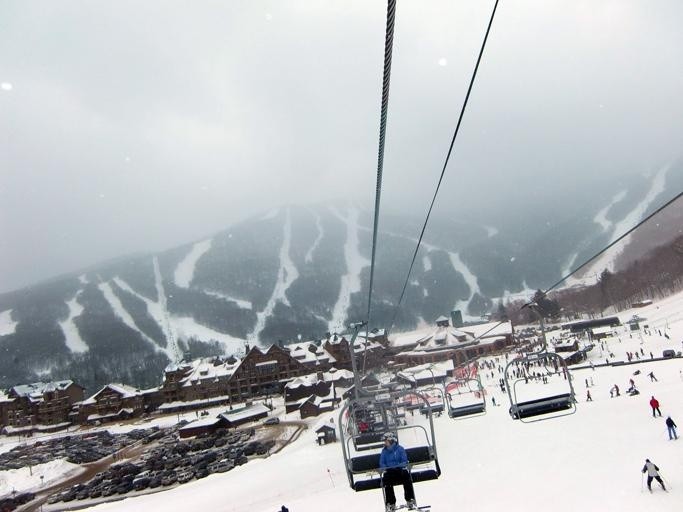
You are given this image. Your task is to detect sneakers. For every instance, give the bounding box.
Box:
[385,503,395,512]
[405,499,419,511]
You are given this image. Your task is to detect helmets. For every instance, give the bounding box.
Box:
[380,432,398,442]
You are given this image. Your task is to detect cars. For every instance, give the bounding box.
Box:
[0,416,279,512]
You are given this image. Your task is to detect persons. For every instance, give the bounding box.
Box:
[641,458,666,493]
[650,395,661,417]
[486,328,670,406]
[380,432,417,512]
[666,415,678,440]
